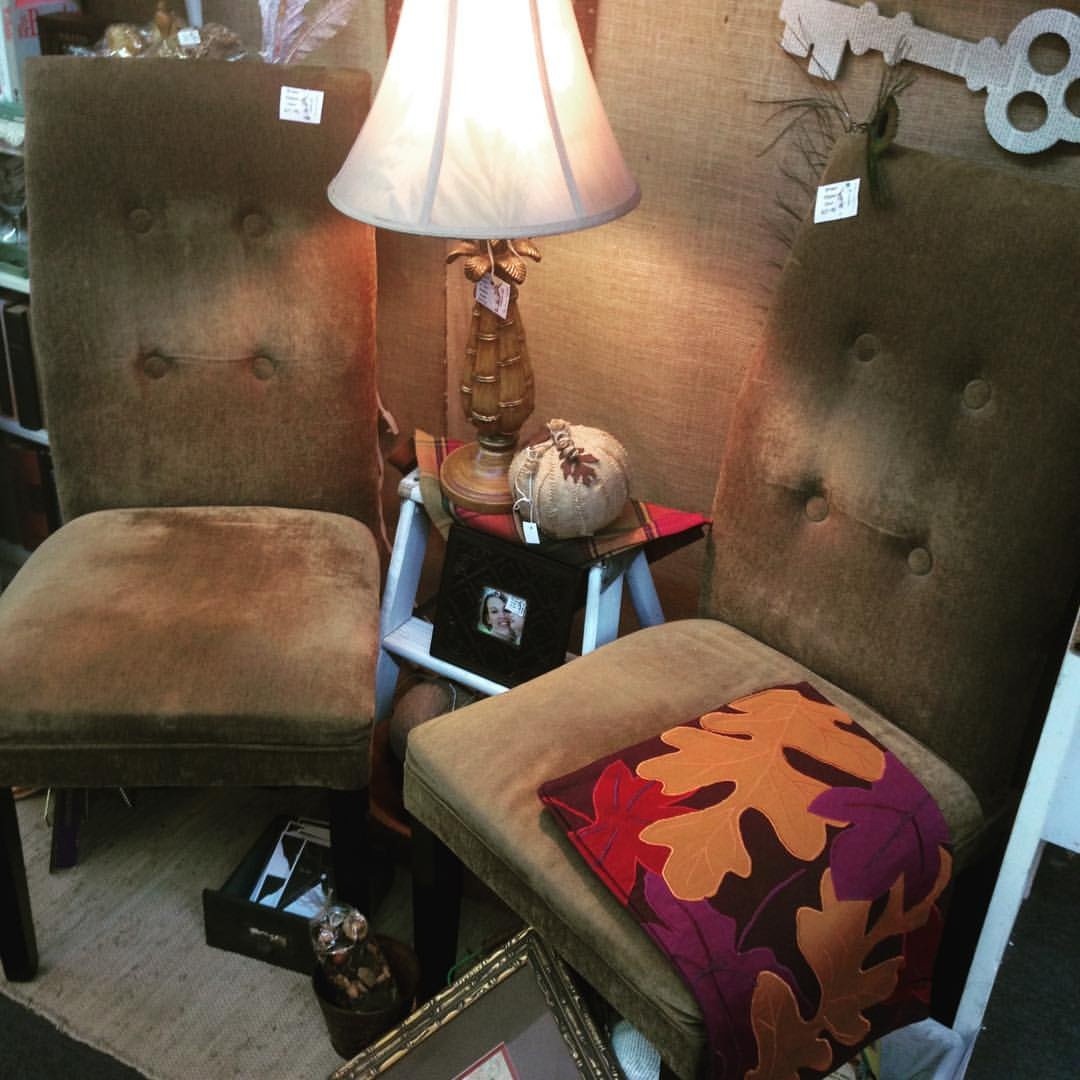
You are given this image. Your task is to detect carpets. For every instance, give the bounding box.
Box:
[0,778,533,1080]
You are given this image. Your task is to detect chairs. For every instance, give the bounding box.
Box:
[0,50,422,980]
[398,127,1080,1080]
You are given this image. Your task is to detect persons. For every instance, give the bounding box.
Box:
[482,591,523,646]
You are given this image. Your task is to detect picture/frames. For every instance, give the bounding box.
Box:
[319,914,627,1080]
[424,527,582,694]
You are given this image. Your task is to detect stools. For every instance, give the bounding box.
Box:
[355,460,723,760]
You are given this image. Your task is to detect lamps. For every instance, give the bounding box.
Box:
[326,0,649,518]
